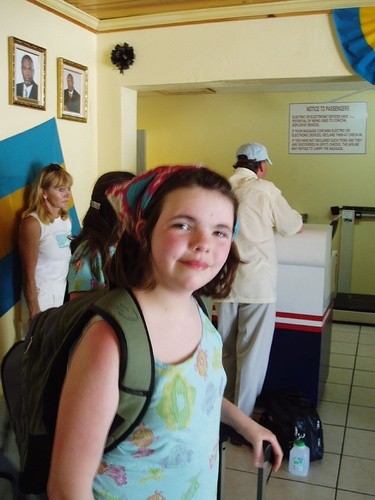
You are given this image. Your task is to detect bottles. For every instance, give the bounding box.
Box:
[288,441,310,476]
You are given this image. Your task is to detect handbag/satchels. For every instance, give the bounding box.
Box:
[259,389,323,463]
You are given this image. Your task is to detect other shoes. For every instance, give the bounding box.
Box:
[220,423,246,448]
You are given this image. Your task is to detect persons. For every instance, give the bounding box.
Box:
[217,143,302,446]
[63,74,81,113]
[21,163,136,339]
[47,165,283,500]
[16,55,37,99]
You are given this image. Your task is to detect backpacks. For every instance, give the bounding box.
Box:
[0,287,155,492]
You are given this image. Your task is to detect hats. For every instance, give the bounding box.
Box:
[235,141,273,166]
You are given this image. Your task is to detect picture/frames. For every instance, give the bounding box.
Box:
[56,57,88,124]
[8,36,48,111]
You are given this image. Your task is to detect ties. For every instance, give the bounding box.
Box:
[25,89,28,98]
[68,91,72,101]
